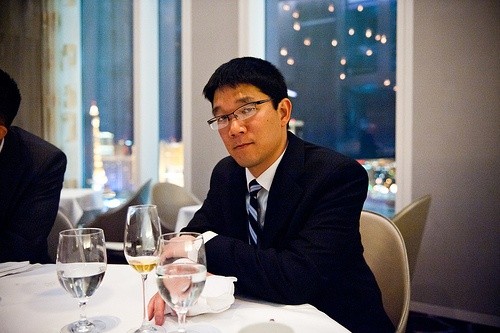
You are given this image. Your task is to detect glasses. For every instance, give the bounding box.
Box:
[206,98,275,131]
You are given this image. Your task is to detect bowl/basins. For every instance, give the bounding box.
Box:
[236,322,295,333]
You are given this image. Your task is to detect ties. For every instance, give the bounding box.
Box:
[247,179,263,248]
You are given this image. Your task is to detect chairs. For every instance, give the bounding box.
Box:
[49,212,84,263]
[83,177,152,248]
[359,209,412,333]
[392,195,433,284]
[152,183,202,230]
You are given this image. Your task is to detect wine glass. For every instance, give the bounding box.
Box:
[124,204,166,333]
[153,232,207,333]
[56,227,107,333]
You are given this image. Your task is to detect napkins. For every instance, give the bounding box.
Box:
[1,261,43,277]
[164,275,237,316]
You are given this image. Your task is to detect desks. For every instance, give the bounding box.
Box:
[174,204,202,233]
[63,188,103,227]
[0,261,352,333]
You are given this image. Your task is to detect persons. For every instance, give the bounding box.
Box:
[0,67,67,264]
[147,56,398,333]
